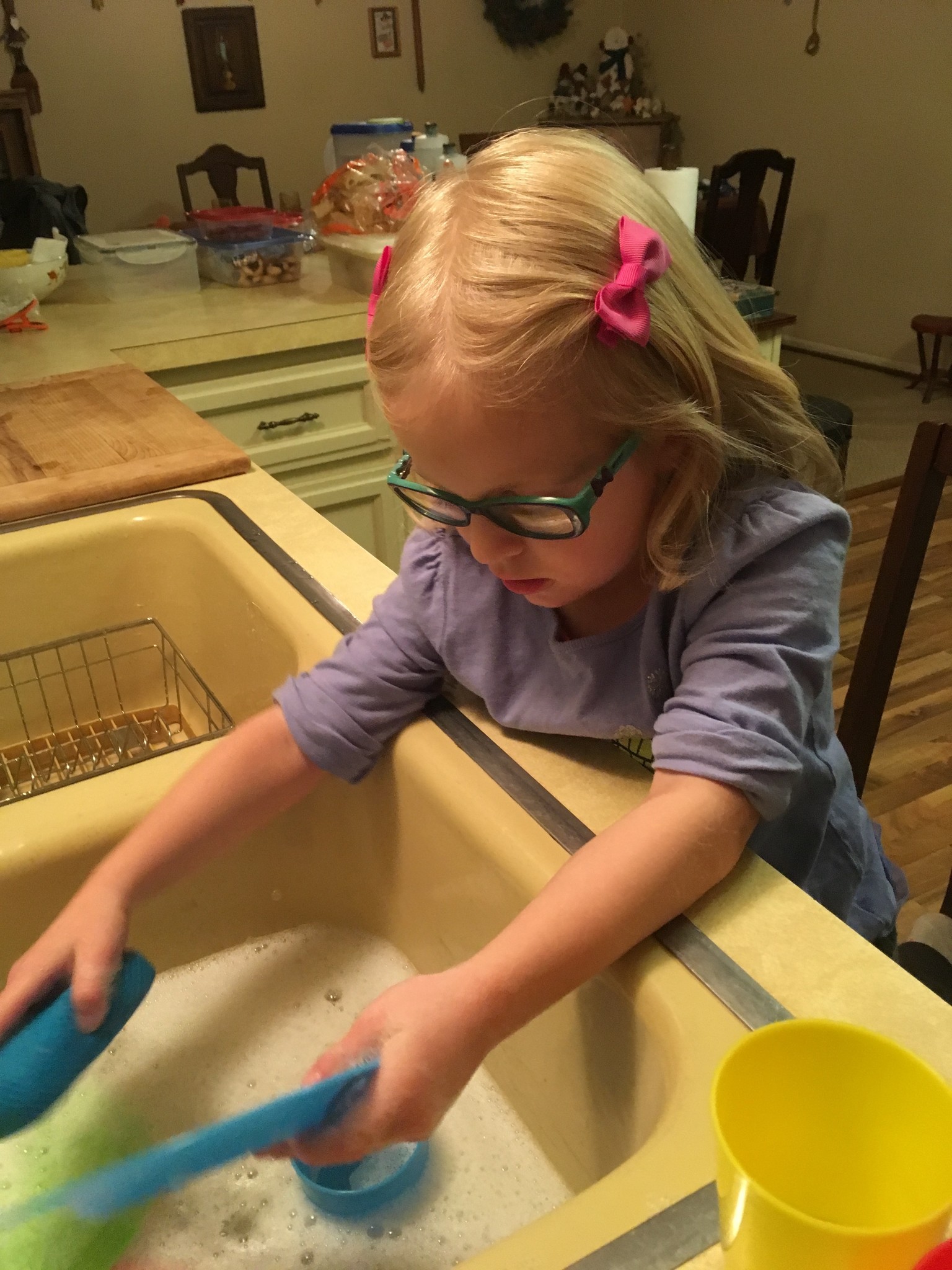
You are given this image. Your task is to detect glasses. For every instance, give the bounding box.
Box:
[384,430,636,540]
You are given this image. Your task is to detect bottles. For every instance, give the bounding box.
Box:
[400,121,468,180]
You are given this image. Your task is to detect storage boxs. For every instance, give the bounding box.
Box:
[73,220,396,305]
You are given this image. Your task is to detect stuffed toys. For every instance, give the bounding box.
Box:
[553,26,652,117]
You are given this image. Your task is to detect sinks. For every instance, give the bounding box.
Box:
[1,723,757,1269]
[0,497,347,807]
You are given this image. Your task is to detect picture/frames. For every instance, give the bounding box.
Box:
[368,7,402,59]
[182,6,266,115]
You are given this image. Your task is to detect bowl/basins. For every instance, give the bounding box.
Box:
[1,253,68,301]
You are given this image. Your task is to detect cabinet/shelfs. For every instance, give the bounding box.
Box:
[539,114,680,172]
[147,339,415,571]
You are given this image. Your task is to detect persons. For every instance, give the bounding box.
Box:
[0,129,908,1163]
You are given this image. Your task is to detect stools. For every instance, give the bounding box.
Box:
[903,314,952,405]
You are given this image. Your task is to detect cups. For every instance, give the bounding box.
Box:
[709,1019,952,1270]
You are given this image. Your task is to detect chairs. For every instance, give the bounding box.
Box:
[177,144,274,213]
[701,148,796,286]
[838,421,952,1005]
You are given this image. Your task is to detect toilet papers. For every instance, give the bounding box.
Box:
[645,165,699,235]
